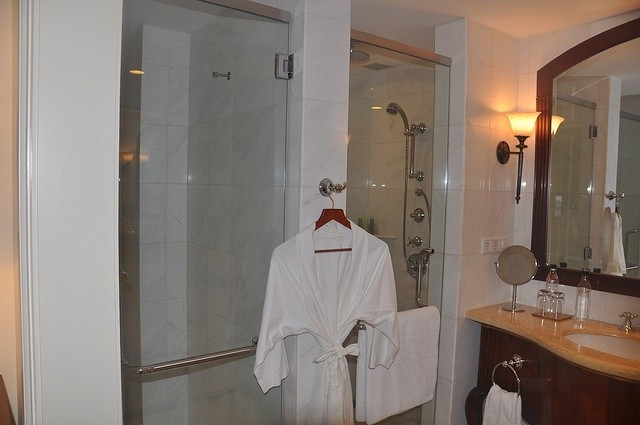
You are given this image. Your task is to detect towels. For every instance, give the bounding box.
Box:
[356,307,442,424]
[604,211,626,276]
[481,385,521,425]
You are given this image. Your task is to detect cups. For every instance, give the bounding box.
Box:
[549,292,566,317]
[537,291,549,316]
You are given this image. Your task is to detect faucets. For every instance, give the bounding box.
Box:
[618,311,638,334]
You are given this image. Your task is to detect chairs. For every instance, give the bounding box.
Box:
[0,374,16,425]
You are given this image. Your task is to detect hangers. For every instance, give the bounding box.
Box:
[311,183,352,253]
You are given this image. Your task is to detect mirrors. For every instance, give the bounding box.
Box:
[530,16,640,298]
[495,245,541,284]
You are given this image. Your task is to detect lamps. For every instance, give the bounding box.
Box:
[551,114,565,135]
[496,111,540,203]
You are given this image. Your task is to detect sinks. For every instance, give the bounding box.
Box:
[562,329,640,362]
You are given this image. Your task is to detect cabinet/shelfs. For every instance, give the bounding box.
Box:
[491,332,611,425]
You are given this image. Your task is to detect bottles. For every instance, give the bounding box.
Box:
[544,264,560,314]
[572,268,592,324]
